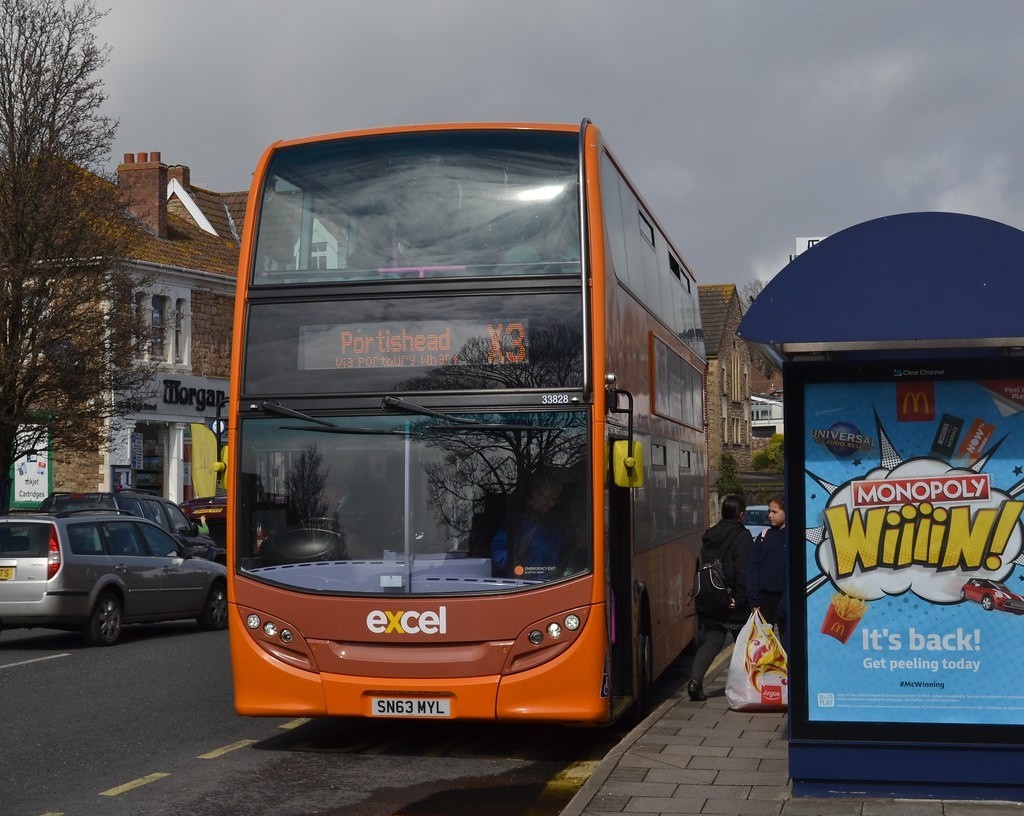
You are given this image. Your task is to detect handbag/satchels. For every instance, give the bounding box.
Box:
[725,608,788,710]
[691,559,735,617]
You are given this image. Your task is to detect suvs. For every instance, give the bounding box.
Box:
[0,509,231,648]
[38,489,285,565]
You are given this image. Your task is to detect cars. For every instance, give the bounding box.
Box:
[744,504,775,542]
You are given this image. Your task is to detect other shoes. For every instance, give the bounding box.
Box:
[688,678,707,701]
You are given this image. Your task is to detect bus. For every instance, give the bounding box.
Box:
[189,115,710,730]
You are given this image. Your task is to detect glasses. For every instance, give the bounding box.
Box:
[537,491,559,502]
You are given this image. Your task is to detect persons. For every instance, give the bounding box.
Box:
[688,495,754,700]
[491,473,562,583]
[745,494,788,653]
